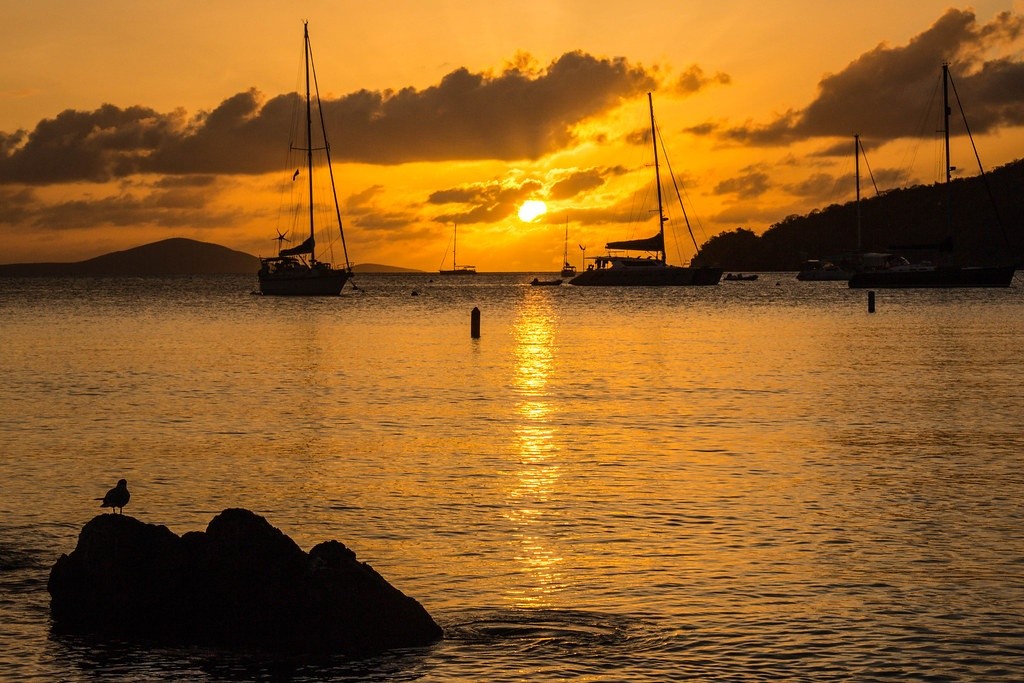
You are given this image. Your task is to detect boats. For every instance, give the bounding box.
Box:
[724,275,758,281]
[530,279,562,286]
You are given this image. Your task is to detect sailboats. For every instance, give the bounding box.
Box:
[258,20,355,296]
[847,61,1018,288]
[569,93,726,286]
[562,215,577,278]
[438,223,478,274]
[796,135,911,280]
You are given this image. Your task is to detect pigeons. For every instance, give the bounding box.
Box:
[100,479,131,514]
